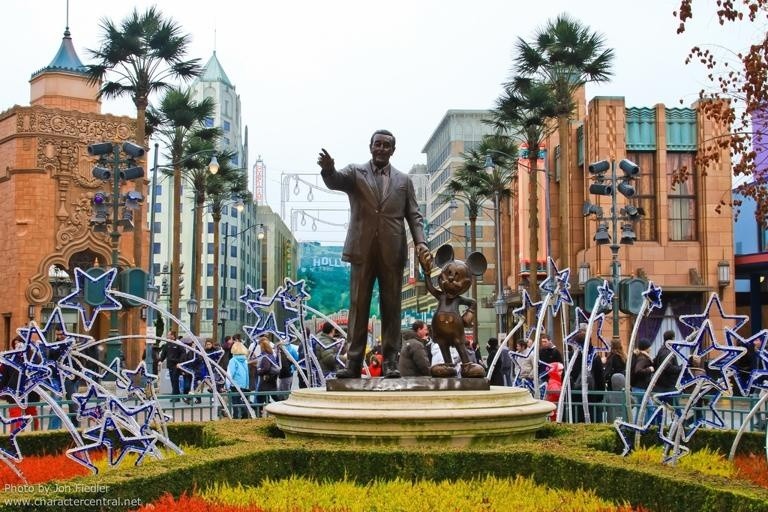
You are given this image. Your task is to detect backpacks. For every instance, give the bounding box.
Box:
[631,352,651,370]
[573,351,600,391]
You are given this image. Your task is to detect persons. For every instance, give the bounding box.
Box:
[316,128,435,378]
[0,335,43,434]
[46,322,87,428]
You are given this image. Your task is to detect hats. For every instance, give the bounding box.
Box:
[637,338,651,350]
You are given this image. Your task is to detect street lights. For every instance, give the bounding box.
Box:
[448,148,553,331]
[583,157,645,355]
[149,144,264,345]
[87,141,145,382]
